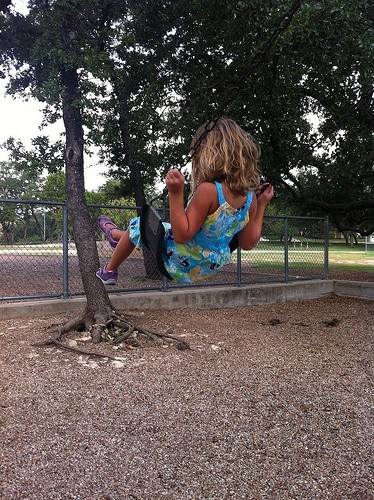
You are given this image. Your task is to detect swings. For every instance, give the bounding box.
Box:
[142,1,374,280]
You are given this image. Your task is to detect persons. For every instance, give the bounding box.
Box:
[95,117,274,285]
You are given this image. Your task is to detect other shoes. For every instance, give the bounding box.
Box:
[97,215,120,252]
[96,263,118,285]
